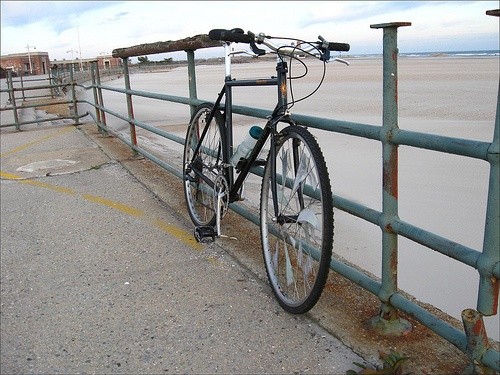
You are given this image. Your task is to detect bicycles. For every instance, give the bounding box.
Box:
[183,27,353,315]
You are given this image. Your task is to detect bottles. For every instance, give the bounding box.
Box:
[229,125,264,167]
